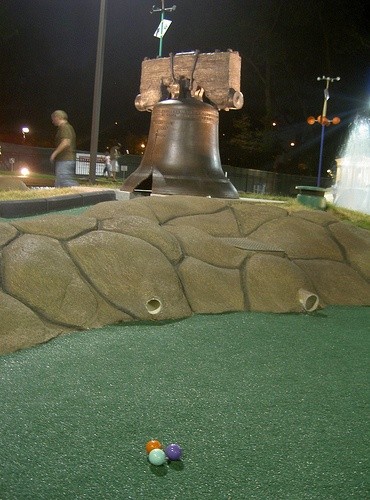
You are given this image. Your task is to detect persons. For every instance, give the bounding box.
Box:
[49,110,80,187]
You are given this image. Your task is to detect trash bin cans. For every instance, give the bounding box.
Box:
[295,186,330,210]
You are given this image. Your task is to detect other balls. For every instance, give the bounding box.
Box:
[149,448,165,466]
[166,444,181,460]
[145,439,163,452]
